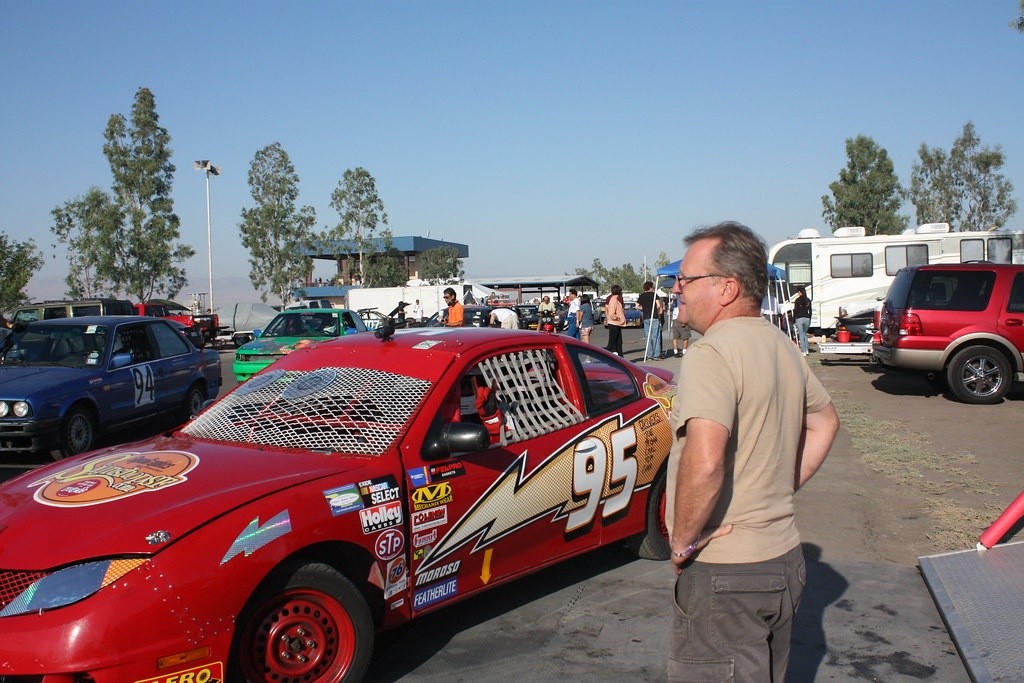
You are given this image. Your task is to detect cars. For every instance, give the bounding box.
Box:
[522,305,572,330]
[323,307,405,335]
[0,316,224,464]
[421,303,497,330]
[233,307,370,383]
[0,324,680,683]
[599,301,644,329]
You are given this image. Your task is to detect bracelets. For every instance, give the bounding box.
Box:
[577,320,581,324]
[671,533,701,557]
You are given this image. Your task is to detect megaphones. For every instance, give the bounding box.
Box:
[193,159,221,175]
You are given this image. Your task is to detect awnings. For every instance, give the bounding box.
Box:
[643,258,799,363]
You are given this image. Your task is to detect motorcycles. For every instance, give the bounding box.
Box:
[538,309,557,332]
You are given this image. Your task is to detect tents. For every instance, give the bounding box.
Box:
[397,277,504,298]
[145,299,192,315]
[209,302,286,332]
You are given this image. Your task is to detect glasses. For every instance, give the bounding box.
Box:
[675,274,730,287]
[443,296,450,299]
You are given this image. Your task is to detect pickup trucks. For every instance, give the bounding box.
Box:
[130,303,221,349]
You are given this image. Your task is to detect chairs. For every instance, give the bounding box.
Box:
[930,283,945,300]
[113,336,131,353]
[306,318,323,329]
[50,338,72,362]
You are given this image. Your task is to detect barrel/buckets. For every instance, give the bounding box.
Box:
[837,331,851,342]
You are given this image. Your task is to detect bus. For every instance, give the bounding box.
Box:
[765,221,1024,348]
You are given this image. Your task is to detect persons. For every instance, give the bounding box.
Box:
[665,221,839,683]
[414,300,423,323]
[794,286,812,356]
[537,296,556,333]
[563,289,582,341]
[152,312,182,317]
[673,295,692,357]
[489,308,520,330]
[204,309,216,348]
[605,285,627,358]
[578,294,594,363]
[636,281,665,361]
[398,301,407,323]
[441,288,464,327]
[315,272,362,286]
[490,291,505,299]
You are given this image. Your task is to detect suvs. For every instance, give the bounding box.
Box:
[7,296,135,352]
[871,259,1024,403]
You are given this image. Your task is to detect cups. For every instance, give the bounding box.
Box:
[821,335,826,343]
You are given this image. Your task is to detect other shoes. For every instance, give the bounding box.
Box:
[674,353,679,358]
[801,351,808,356]
